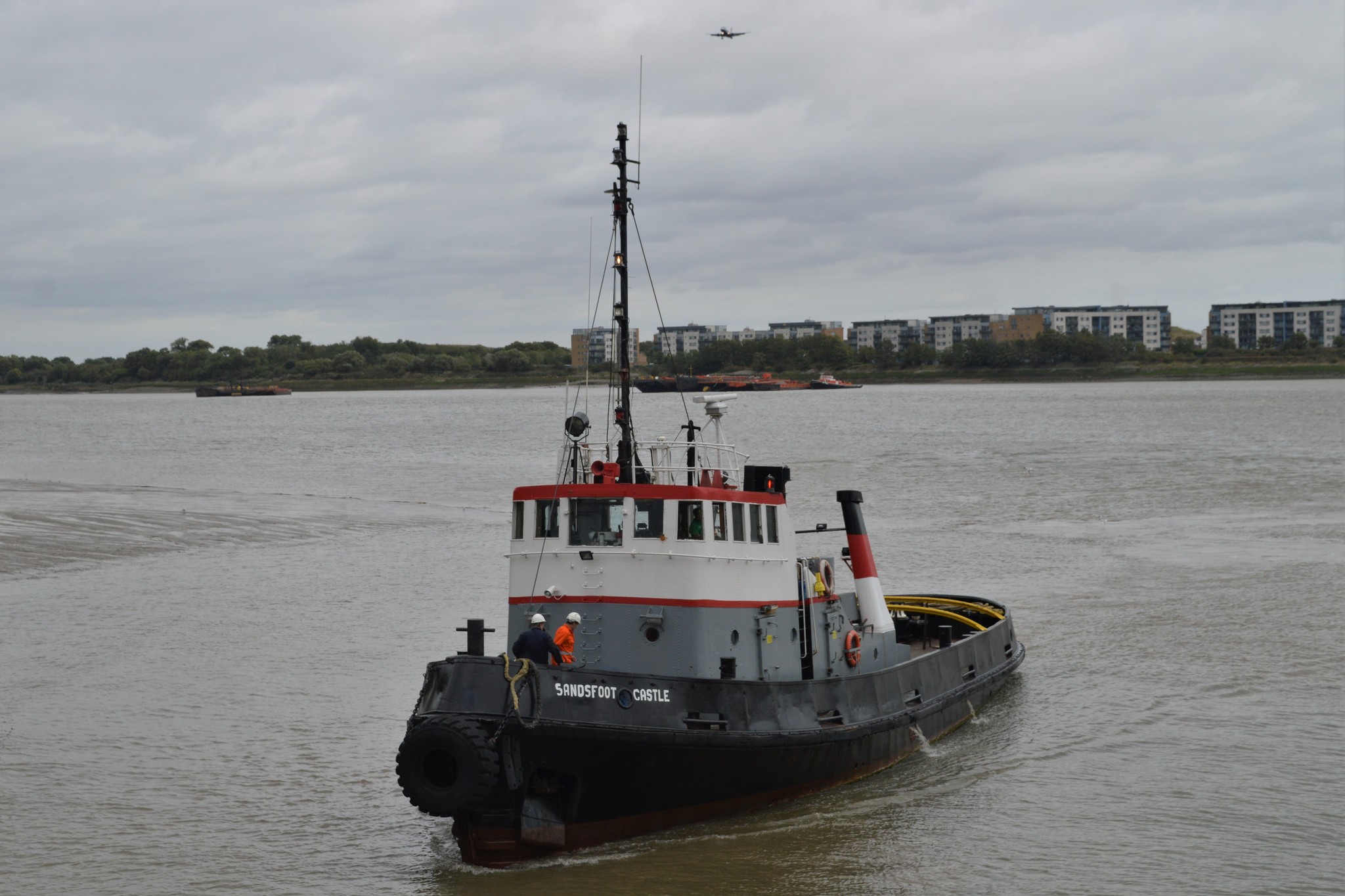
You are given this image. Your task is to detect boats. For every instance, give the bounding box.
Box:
[195,381,292,397]
[631,366,810,393]
[810,372,863,389]
[397,54,1026,868]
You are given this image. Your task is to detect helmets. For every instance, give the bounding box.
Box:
[566,612,582,624]
[531,613,546,623]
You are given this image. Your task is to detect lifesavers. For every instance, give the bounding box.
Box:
[395,713,499,816]
[819,559,835,595]
[846,630,861,666]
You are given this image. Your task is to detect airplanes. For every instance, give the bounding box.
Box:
[710,29,746,39]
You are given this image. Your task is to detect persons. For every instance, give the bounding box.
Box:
[551,612,581,665]
[512,614,563,666]
[689,508,704,539]
